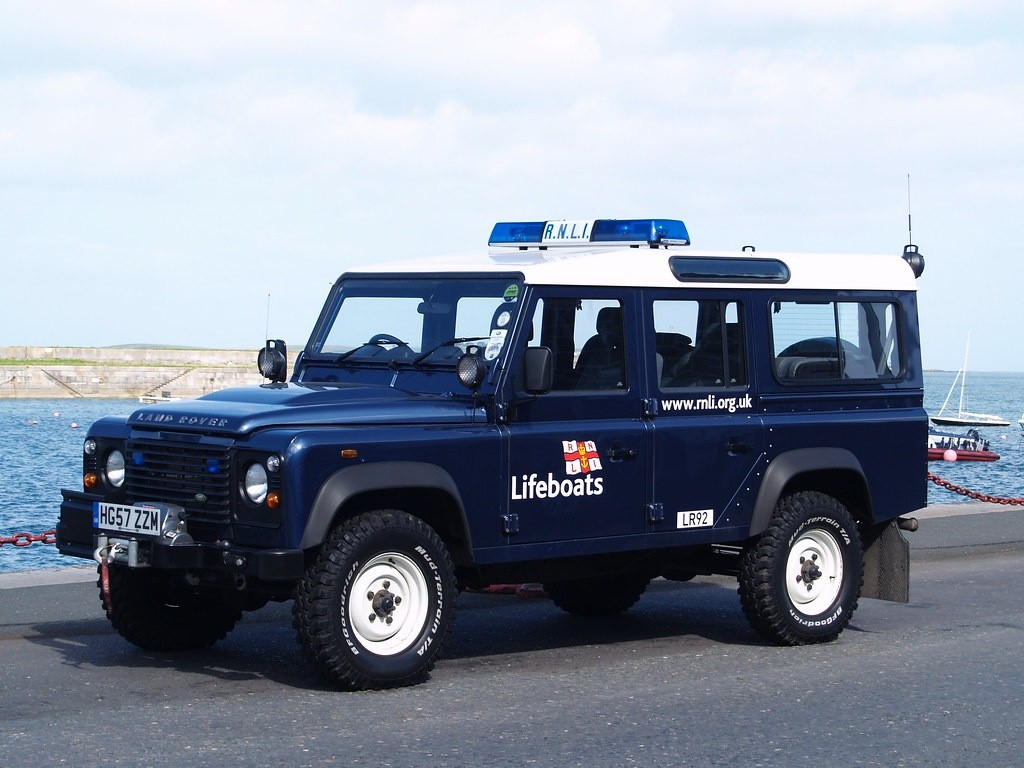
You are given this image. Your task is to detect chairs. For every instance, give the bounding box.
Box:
[475,302,535,360]
[688,320,746,388]
[658,330,692,386]
[575,308,627,389]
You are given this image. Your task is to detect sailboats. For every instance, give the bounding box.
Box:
[930,325,1012,425]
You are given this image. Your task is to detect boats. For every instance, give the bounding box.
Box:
[1018,413,1024,431]
[927,425,1001,462]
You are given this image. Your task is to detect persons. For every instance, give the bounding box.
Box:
[929,436,990,451]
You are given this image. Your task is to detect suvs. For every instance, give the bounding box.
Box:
[57,217,933,692]
